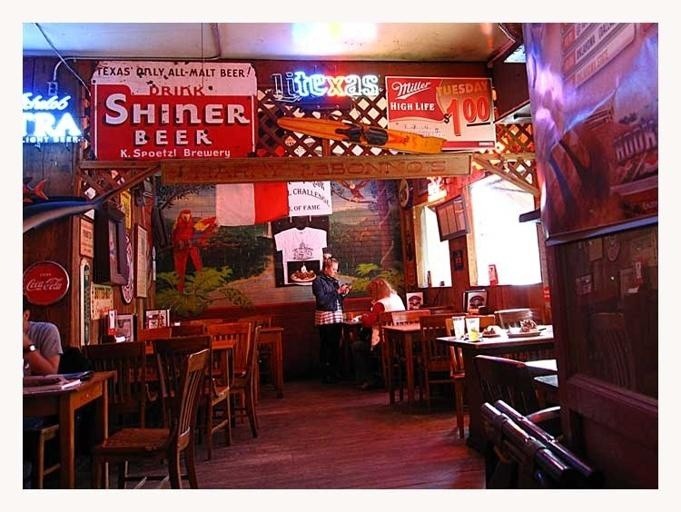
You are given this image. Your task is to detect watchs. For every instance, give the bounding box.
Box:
[23,343,37,354]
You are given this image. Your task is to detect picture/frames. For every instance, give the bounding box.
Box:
[135,224,149,298]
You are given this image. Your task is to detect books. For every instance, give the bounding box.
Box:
[24,378,81,391]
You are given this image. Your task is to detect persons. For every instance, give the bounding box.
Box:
[351,277,407,391]
[23,295,64,377]
[312,257,354,385]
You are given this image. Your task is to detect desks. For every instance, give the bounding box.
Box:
[24,368,117,488]
[90,339,238,444]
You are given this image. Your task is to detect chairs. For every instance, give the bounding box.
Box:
[26,420,62,489]
[342,295,546,440]
[150,334,237,450]
[84,339,150,422]
[96,347,211,491]
[170,309,284,438]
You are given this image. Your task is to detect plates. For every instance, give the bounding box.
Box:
[507,331,541,338]
[482,333,502,338]
[291,271,317,283]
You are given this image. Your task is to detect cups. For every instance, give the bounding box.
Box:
[466,318,480,342]
[451,315,466,341]
